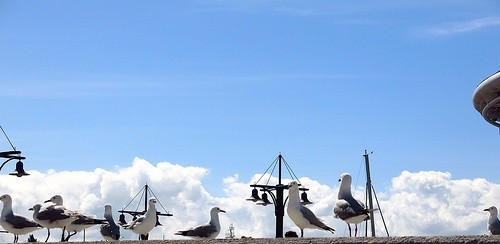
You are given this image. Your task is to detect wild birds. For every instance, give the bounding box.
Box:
[174,207,226,240]
[333,173,379,237]
[44,194,109,242]
[100,204,121,241]
[29,204,86,242]
[286,180,335,238]
[483,206,500,235]
[0,194,43,244]
[118,197,157,241]
